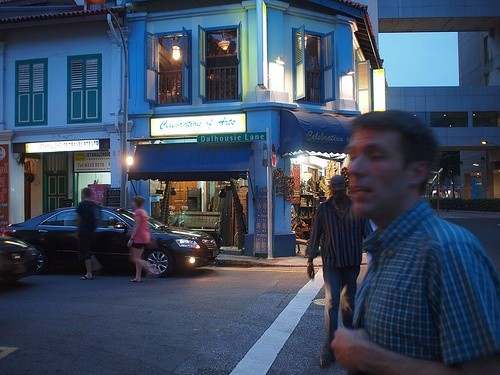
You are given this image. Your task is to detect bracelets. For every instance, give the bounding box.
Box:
[130,238,134,240]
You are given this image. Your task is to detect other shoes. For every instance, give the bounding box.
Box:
[319,354,335,368]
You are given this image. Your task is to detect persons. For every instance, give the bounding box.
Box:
[306,175,374,368]
[332,109,500,375]
[76,189,104,280]
[127,196,154,282]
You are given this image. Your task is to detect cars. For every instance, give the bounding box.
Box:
[0,234,45,282]
[0,205,220,279]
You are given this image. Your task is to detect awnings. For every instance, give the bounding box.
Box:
[279,107,357,157]
[128,143,250,181]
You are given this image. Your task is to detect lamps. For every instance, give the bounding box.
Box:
[169,187,177,195]
[171,43,180,59]
[218,187,228,199]
[16,153,26,164]
[345,67,356,75]
[217,35,231,50]
[275,56,286,65]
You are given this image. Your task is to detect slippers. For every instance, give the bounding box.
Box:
[130,279,142,283]
[80,276,94,280]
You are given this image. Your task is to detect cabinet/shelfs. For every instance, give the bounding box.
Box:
[298,195,326,239]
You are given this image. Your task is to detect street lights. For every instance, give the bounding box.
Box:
[83,0,135,208]
